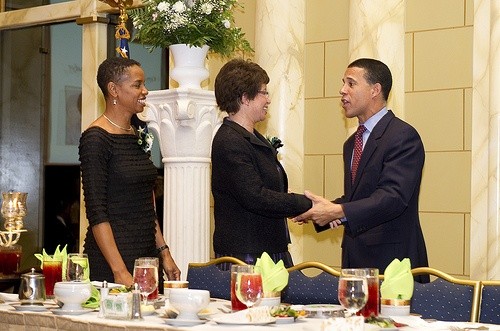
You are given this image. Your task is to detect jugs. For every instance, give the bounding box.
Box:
[19,267,47,304]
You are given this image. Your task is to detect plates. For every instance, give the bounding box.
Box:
[49,308,94,315]
[212,313,277,326]
[164,319,207,327]
[12,304,47,312]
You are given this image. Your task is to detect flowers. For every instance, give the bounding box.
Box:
[265,133,284,161]
[137,126,154,153]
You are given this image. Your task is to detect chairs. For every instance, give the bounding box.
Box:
[185,256,500,325]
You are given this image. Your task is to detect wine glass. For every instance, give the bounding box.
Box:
[133,259,158,305]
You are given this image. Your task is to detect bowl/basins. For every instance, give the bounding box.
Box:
[169,289,210,319]
[53,281,92,309]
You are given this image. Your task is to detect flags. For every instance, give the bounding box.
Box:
[115,2,131,59]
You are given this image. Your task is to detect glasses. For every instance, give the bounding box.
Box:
[256,90,268,96]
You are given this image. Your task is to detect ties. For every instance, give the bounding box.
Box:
[351,125,367,187]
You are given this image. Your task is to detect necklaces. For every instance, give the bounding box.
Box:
[102,113,132,131]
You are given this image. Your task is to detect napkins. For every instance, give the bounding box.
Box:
[254,252,289,292]
[380,257,414,300]
[34,244,69,283]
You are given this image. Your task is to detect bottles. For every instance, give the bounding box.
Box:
[98,280,110,317]
[131,283,143,321]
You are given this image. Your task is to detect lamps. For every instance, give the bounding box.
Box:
[0,190,30,247]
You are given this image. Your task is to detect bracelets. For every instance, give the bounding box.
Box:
[157,244,169,253]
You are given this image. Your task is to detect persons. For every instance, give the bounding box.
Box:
[292,58,431,284]
[210,58,340,273]
[78,57,182,294]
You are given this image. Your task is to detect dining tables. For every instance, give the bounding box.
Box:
[0,300,500,331]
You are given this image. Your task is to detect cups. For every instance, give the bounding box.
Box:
[338,267,411,317]
[231,264,283,313]
[132,256,189,300]
[0,243,90,300]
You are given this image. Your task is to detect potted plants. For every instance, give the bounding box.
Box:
[125,0,256,89]
